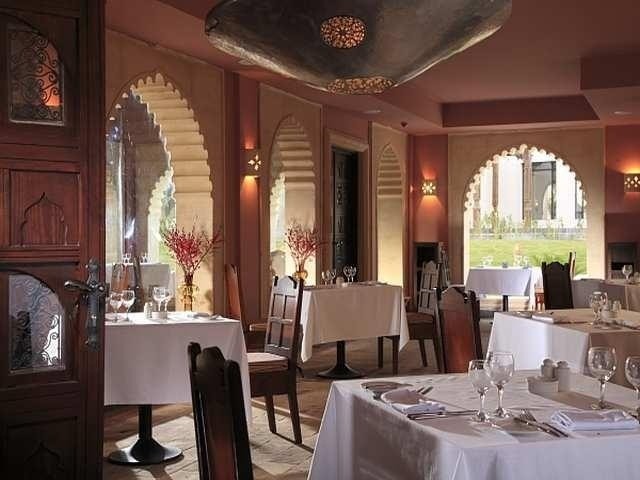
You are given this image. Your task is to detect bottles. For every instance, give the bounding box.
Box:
[143,301,153,319]
[540,359,572,392]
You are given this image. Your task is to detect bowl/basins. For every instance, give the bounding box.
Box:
[151,310,167,321]
[526,376,559,395]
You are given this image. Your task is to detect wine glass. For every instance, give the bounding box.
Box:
[586,346,618,410]
[320,265,357,287]
[467,360,494,424]
[621,265,634,284]
[110,286,174,323]
[587,290,608,328]
[624,355,640,417]
[483,350,516,419]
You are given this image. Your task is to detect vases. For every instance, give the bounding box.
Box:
[176,275,200,310]
[292,264,308,281]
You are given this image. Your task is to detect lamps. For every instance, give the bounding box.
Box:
[242,148,265,178]
[421,179,439,196]
[623,173,640,193]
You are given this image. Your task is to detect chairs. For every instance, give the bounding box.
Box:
[246,274,305,445]
[186,341,254,479]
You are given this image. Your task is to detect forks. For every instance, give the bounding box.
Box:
[519,408,570,440]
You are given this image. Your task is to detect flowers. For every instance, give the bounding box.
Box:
[158,212,226,311]
[283,217,325,266]
